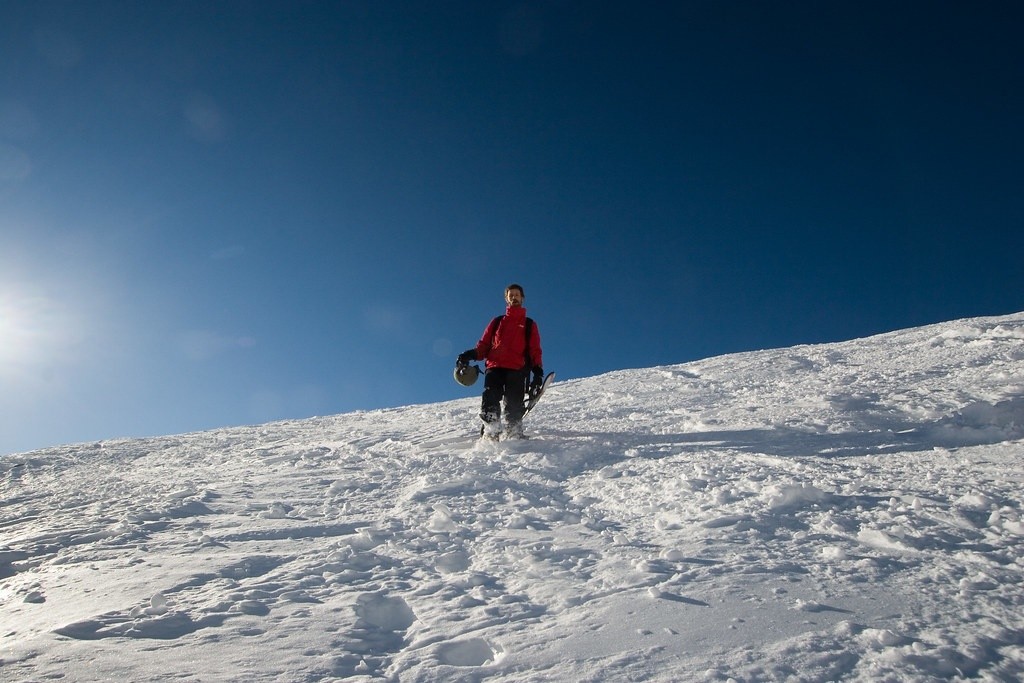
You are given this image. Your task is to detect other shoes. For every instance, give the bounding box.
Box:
[506,426,530,440]
[482,421,499,441]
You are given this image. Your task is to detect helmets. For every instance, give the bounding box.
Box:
[453,360,479,387]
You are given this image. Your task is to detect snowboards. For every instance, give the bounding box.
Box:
[522,371,555,420]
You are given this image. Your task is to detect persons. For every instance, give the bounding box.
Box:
[455,284,544,441]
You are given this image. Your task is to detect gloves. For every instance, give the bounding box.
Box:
[458,349,474,364]
[531,375,542,390]
